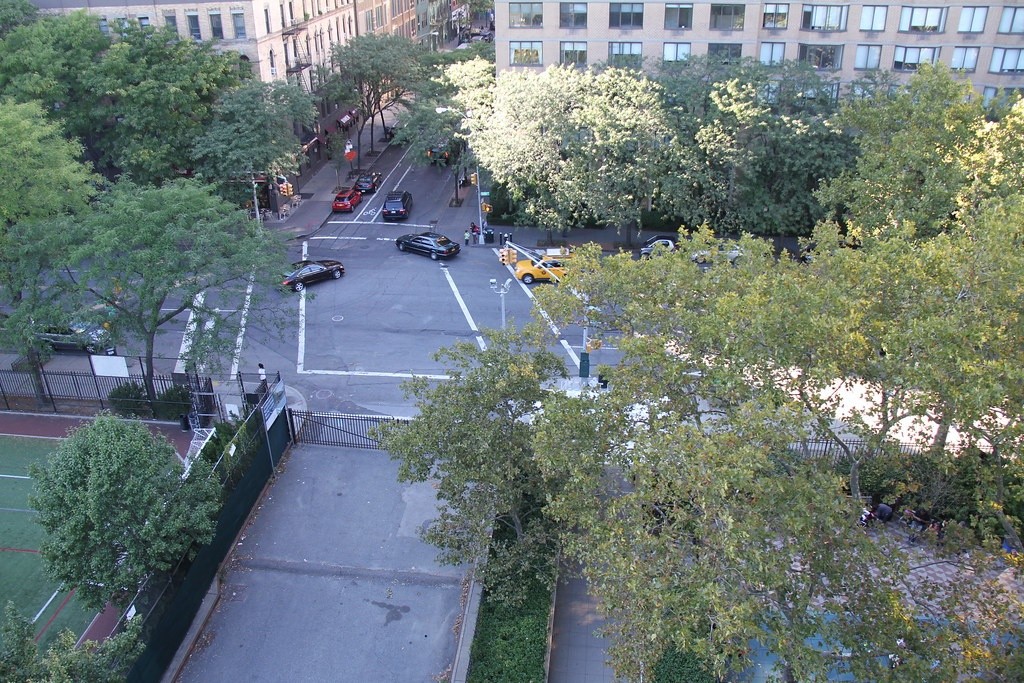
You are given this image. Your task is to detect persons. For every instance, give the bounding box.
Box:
[470,222,478,241]
[258,363,269,390]
[908,520,970,563]
[464,230,470,246]
[860,504,873,534]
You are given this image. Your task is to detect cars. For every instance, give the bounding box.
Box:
[690,240,750,267]
[514,258,571,286]
[30,314,113,354]
[425,135,460,165]
[354,171,382,193]
[396,231,462,261]
[639,234,688,261]
[457,21,494,52]
[331,188,363,212]
[275,258,346,292]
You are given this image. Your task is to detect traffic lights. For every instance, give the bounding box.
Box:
[486,204,493,214]
[585,342,593,353]
[470,173,477,186]
[499,248,517,266]
[279,182,294,198]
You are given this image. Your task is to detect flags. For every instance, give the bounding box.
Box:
[344,141,356,161]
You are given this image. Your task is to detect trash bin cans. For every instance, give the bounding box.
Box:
[580,353,590,377]
[180,412,201,433]
[484,227,495,243]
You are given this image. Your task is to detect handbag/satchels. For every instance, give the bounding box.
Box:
[474,226,479,232]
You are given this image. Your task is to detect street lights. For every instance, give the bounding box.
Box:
[435,107,485,244]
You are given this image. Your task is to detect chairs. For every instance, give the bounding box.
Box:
[239,195,301,221]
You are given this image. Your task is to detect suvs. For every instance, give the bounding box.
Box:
[381,190,414,222]
